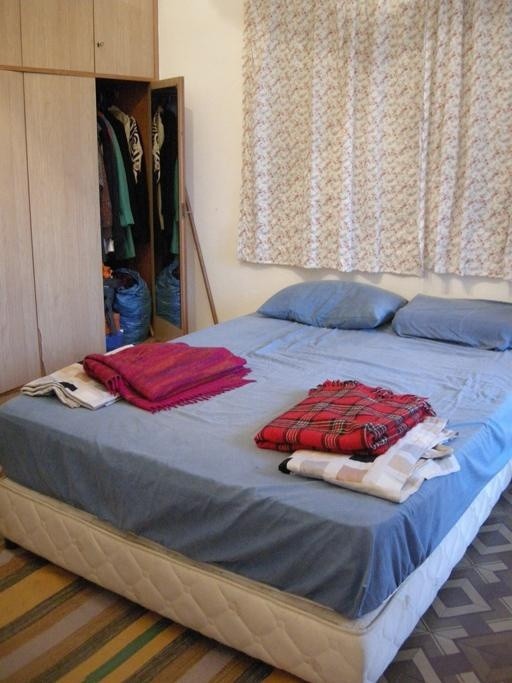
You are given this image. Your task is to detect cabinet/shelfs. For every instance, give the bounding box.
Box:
[20,0,159,79]
[0,0,43,394]
[22,72,190,374]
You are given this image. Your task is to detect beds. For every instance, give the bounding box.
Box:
[1,279,512,683]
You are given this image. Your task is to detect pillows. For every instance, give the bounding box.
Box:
[254,280,408,330]
[391,291,512,353]
